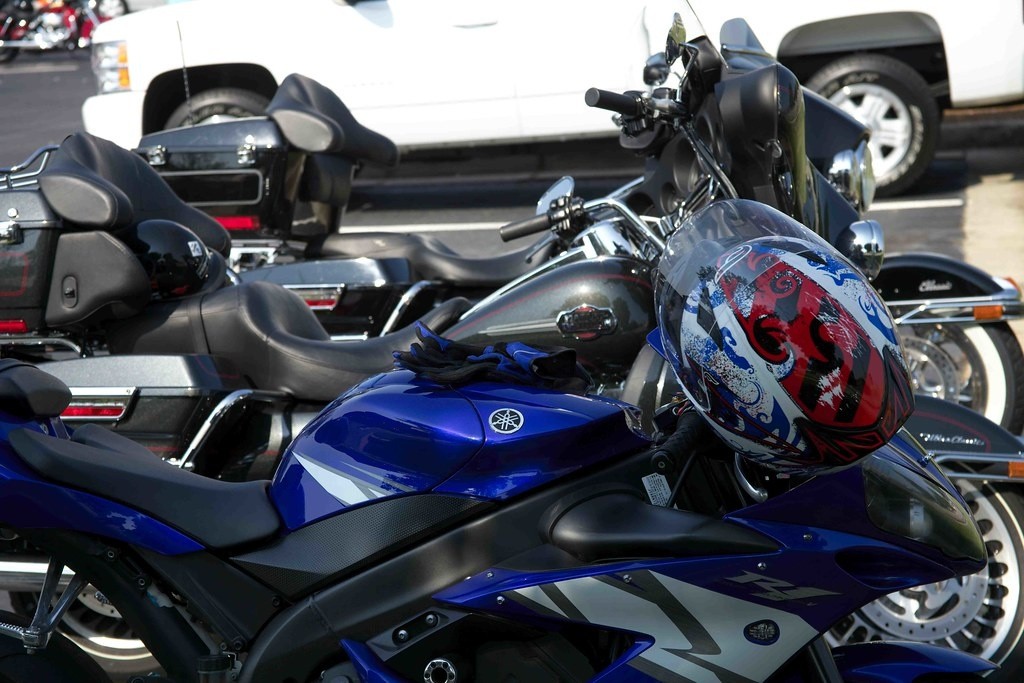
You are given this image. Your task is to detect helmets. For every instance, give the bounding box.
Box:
[648,199,914,474]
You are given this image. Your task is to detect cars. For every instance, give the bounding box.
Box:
[82,0,1024,234]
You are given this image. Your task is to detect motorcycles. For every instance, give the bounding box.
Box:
[0,0,130,64]
[0,12,1024,683]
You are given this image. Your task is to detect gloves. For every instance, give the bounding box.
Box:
[393,322,600,398]
